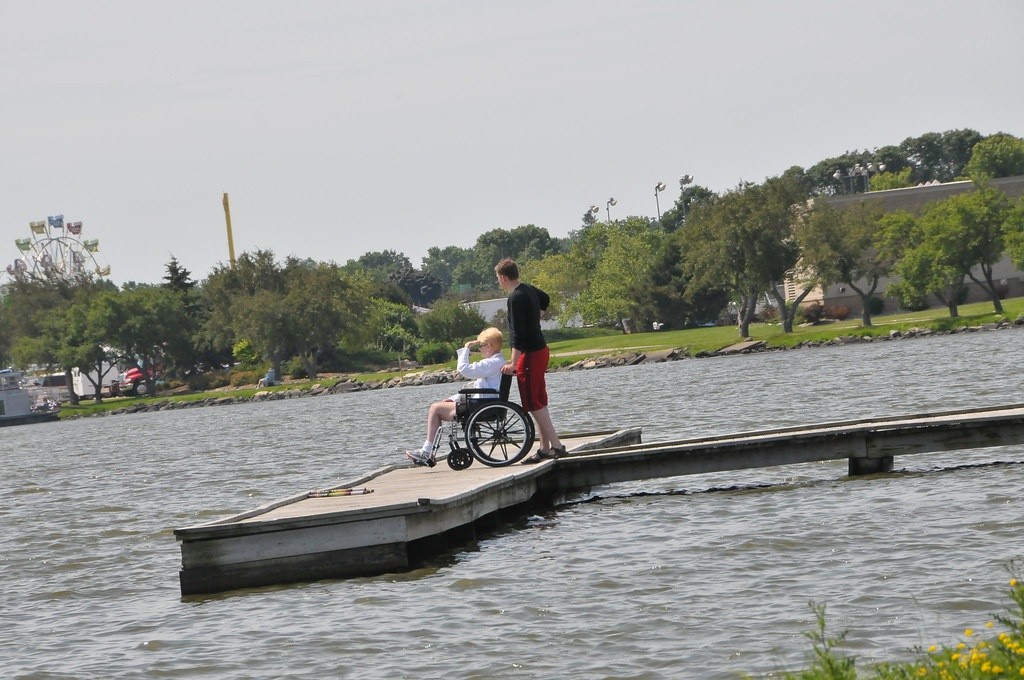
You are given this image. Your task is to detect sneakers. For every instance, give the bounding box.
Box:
[406,449,429,462]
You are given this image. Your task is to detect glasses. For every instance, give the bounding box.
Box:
[479,344,487,348]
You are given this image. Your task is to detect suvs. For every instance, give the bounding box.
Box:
[117,342,169,396]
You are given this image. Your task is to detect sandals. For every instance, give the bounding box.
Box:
[545,445,569,459]
[521,449,554,464]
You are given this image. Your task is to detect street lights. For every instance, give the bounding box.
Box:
[655,181,667,220]
[606,197,618,225]
[587,203,599,221]
[679,174,694,219]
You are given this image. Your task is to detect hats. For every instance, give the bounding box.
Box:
[268,368,274,371]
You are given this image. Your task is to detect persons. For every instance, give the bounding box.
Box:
[256,368,276,390]
[495,260,569,465]
[406,327,506,462]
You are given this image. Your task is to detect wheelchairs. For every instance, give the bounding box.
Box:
[410,368,537,468]
